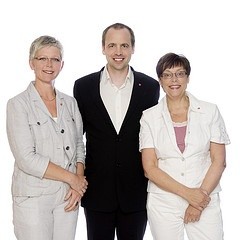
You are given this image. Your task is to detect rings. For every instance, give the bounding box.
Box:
[74,200,79,206]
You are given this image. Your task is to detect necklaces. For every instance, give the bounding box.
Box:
[41,95,57,101]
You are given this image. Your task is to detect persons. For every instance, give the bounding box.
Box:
[6,35,88,240]
[72,22,160,240]
[138,52,231,240]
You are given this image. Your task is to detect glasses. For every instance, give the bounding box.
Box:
[34,56,60,62]
[160,73,189,79]
